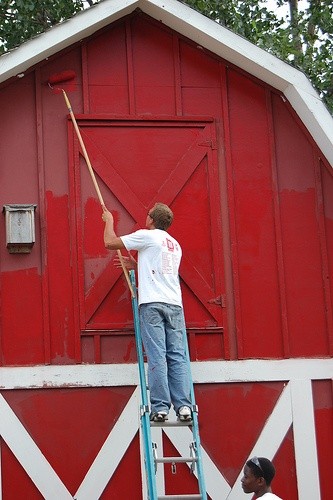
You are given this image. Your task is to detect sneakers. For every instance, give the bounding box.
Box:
[154,410,168,422]
[177,406,192,421]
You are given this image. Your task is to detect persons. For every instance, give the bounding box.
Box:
[101,201,193,424]
[240,456,283,500]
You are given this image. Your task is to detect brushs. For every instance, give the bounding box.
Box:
[45,70,136,299]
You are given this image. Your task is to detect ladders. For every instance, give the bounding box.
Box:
[128,268,207,499]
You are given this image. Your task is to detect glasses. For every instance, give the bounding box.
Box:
[251,456,263,473]
